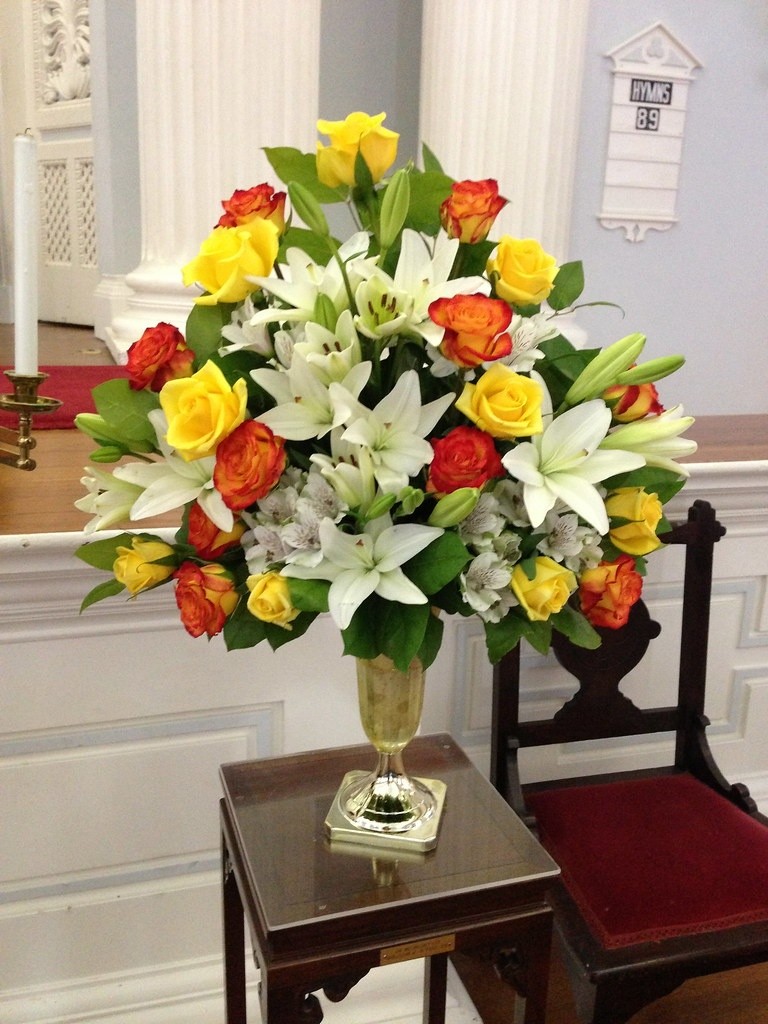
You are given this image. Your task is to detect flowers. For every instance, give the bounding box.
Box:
[69,108,699,674]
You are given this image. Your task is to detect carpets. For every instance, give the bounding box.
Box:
[0,365,129,431]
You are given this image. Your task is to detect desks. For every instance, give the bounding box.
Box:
[217,734,562,1024]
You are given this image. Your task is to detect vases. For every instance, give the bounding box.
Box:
[323,651,447,853]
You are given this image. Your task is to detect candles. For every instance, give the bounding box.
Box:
[13,127,39,375]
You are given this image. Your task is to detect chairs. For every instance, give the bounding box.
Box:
[489,500,768,1024]
